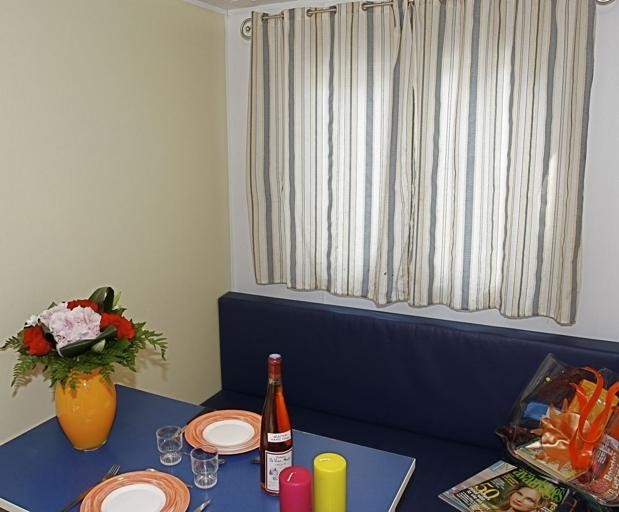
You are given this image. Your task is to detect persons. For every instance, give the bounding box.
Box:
[487,482,545,512]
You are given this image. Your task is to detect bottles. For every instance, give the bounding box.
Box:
[258,353,294,497]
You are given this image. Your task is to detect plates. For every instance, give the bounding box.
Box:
[184,409,263,456]
[79,471,190,512]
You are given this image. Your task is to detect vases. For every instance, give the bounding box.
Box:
[52,365,121,452]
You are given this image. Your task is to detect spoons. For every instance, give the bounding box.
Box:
[184,451,226,465]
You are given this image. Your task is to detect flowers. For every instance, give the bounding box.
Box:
[0,286,169,395]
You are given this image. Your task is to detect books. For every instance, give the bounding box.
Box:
[515,434,594,481]
[436,462,558,512]
[513,434,619,507]
[452,469,571,512]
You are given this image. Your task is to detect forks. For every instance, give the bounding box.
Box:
[61,464,121,512]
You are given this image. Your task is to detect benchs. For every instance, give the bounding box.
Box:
[196,290,619,511]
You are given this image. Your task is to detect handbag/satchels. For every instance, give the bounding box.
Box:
[494,352,619,508]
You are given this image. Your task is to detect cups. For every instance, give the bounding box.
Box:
[155,425,182,466]
[189,445,219,490]
